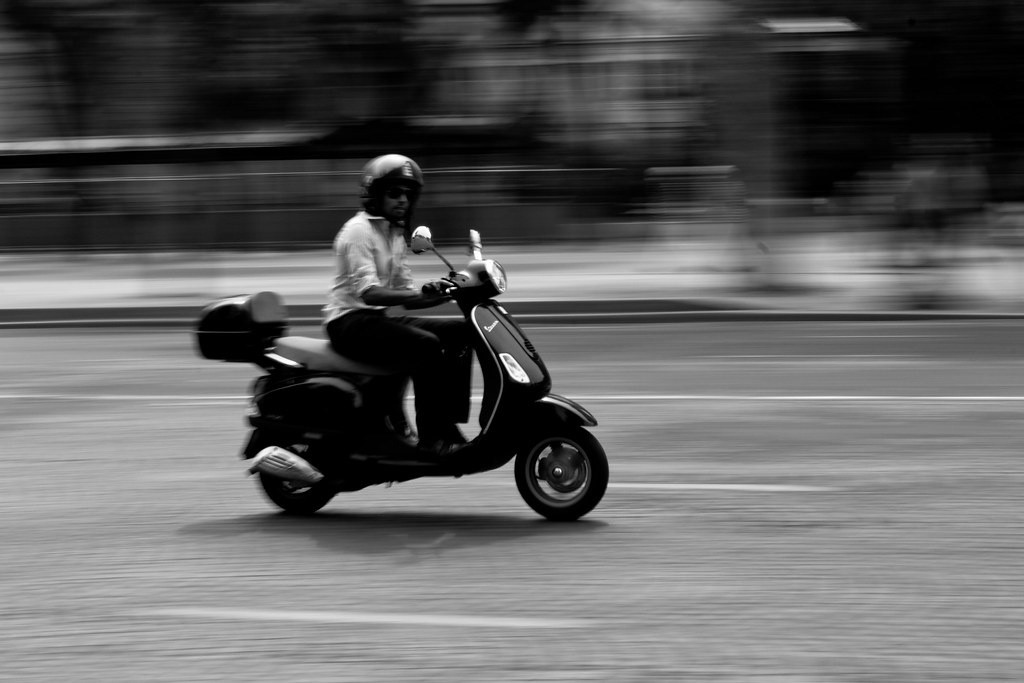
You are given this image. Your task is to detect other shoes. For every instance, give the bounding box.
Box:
[417,439,473,458]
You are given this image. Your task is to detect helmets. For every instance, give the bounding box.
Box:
[359,154,425,218]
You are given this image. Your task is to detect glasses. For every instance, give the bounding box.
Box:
[386,184,417,202]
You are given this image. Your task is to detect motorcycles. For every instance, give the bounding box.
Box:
[193,221,612,534]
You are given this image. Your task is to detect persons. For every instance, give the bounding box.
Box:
[320,152,471,458]
